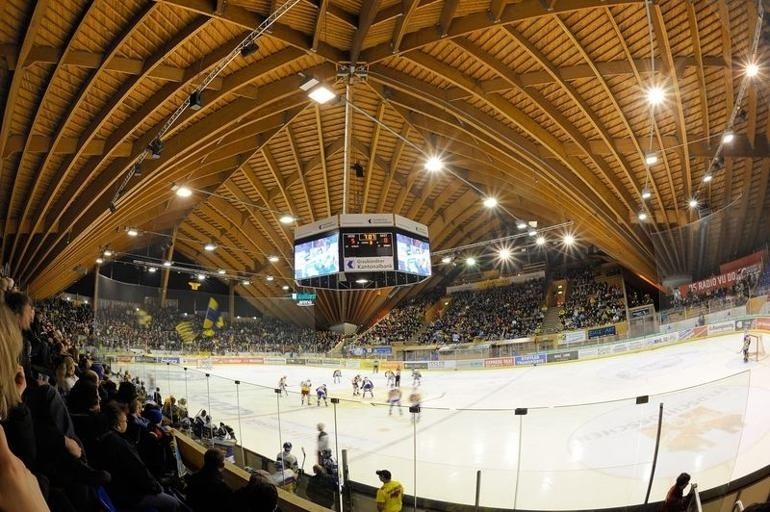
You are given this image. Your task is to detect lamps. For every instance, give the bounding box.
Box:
[293,71,340,106]
[133,36,260,180]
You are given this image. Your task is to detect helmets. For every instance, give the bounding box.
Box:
[283,442,292,451]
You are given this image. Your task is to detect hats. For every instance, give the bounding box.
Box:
[376,470,392,480]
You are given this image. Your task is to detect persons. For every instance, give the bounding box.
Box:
[294,232,338,279]
[381,236,391,247]
[742,501,770,512]
[660,472,698,512]
[349,238,357,246]
[367,234,378,248]
[397,234,431,276]
[742,327,752,363]
[673,271,756,309]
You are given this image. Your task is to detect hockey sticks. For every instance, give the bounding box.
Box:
[301,447,306,470]
[423,393,446,402]
